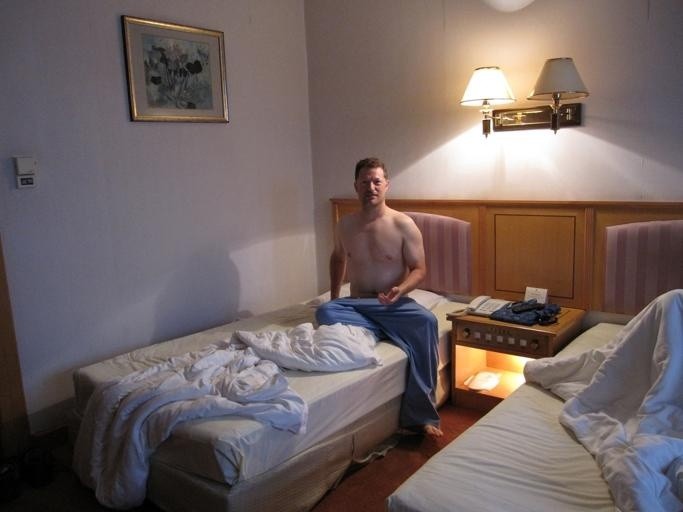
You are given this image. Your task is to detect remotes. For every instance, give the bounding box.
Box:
[512,303,545,314]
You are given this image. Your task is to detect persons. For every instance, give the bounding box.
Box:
[315,157,445,438]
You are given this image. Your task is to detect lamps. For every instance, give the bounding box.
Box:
[459,57,587,138]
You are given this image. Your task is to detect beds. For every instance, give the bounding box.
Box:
[73,212,470,512]
[386,219,683,510]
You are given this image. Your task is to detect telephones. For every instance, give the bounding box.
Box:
[466,296,512,317]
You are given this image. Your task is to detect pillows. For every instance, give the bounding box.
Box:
[301,282,447,311]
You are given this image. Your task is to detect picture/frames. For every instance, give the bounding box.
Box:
[121,15,228,122]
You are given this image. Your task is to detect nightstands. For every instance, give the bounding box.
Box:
[447,306,586,412]
